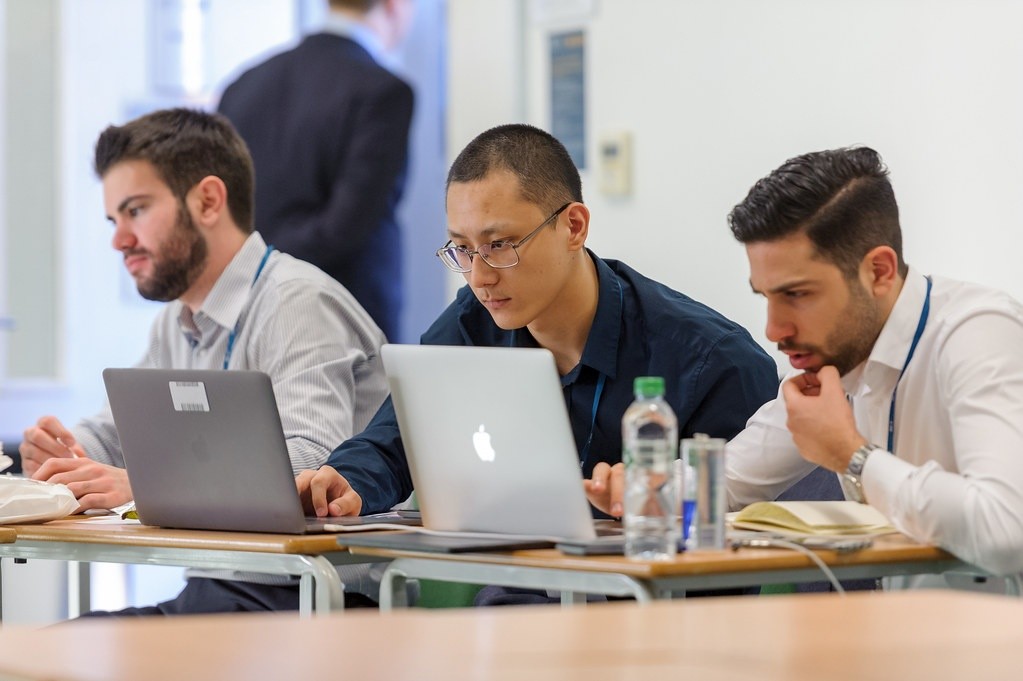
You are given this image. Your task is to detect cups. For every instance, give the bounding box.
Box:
[680,438,726,552]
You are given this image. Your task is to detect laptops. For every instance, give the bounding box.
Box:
[381,344,803,556]
[101,367,419,534]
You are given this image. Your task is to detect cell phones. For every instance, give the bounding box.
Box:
[396,510,421,518]
[555,542,687,557]
[793,537,874,551]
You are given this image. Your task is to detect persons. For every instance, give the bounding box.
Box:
[573,143,1023,601]
[16,109,412,630]
[288,122,780,607]
[215,0,420,382]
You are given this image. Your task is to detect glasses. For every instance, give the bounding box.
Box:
[435,203,574,274]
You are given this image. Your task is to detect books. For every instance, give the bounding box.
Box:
[728,499,900,537]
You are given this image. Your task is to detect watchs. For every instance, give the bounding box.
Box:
[843,441,879,508]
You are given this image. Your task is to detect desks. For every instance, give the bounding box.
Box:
[0,514,1023,681]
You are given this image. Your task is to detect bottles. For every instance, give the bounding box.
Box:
[621,376,677,565]
